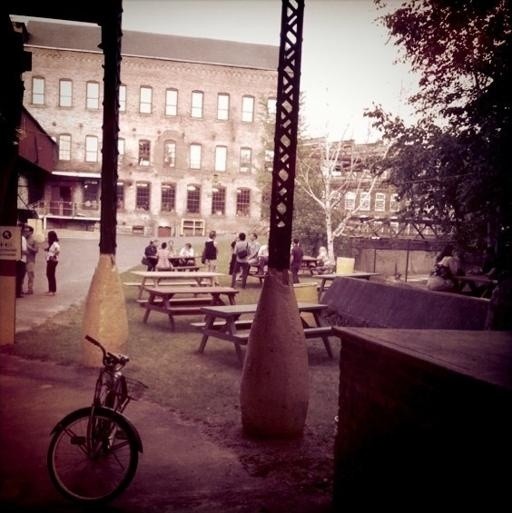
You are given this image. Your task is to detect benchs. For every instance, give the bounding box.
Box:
[314,283,332,290]
[190,321,254,329]
[164,306,201,313]
[125,282,205,286]
[232,326,338,343]
[133,298,212,305]
[149,255,323,284]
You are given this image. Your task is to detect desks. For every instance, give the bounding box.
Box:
[141,286,240,327]
[331,330,510,512]
[313,270,380,303]
[198,302,331,363]
[129,269,229,298]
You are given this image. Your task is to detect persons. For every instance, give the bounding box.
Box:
[316,245,330,265]
[289,237,305,284]
[457,245,502,298]
[228,230,270,289]
[201,229,220,286]
[140,238,195,270]
[13,222,26,300]
[43,229,63,295]
[24,224,39,296]
[426,244,459,290]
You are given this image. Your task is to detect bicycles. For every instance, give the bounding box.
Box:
[43,334,153,504]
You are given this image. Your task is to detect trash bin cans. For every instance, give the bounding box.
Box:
[336,257,355,273]
